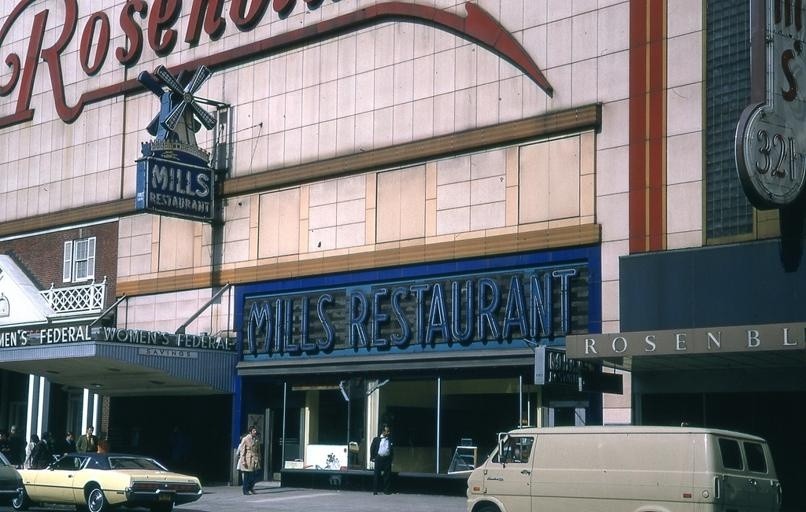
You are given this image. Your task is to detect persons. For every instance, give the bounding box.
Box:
[239,425,262,495]
[235,432,248,483]
[0,424,110,470]
[370,423,393,494]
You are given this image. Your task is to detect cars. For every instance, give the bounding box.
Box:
[17,453,203,512]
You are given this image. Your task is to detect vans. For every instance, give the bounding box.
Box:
[466,425,782,512]
